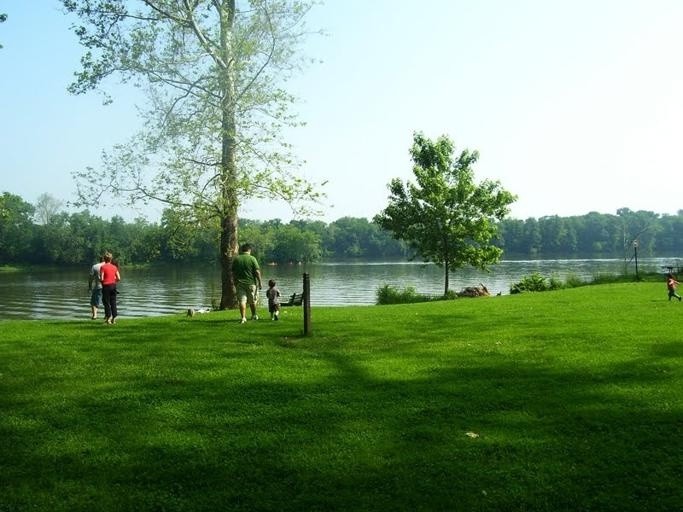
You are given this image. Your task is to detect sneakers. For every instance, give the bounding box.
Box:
[104,317,116,325]
[251,315,259,320]
[271,314,278,321]
[679,296,682,302]
[239,318,247,324]
[92,317,97,320]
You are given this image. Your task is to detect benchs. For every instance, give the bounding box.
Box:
[279,292,303,306]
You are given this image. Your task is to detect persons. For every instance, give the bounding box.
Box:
[231,243,262,325]
[99,251,121,326]
[666,273,682,302]
[266,279,282,321]
[88,256,106,320]
